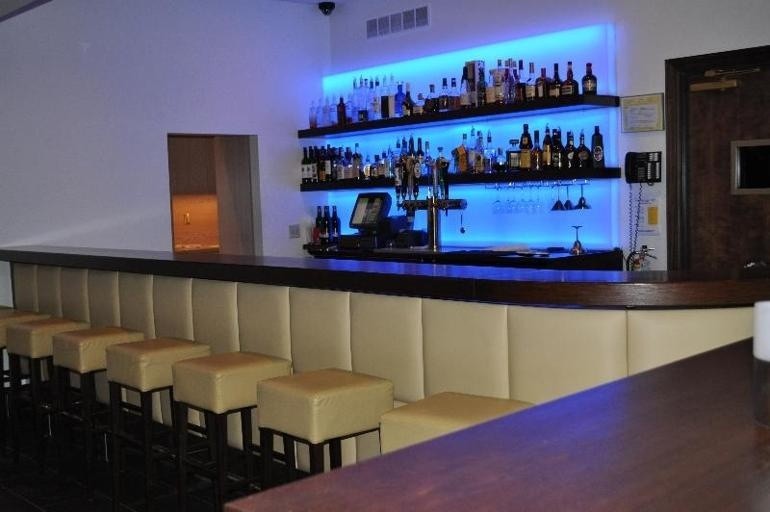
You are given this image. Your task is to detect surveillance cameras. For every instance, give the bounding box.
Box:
[318,2,336,15]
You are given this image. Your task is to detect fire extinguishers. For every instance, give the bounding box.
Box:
[627,247,657,271]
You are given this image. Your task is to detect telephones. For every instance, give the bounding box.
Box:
[625,152,661,183]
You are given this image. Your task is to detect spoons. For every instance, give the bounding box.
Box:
[459,211,465,234]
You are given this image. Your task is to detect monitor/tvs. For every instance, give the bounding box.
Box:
[349,192,392,235]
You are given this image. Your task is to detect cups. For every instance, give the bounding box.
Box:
[312,221,321,243]
[751,300,770,428]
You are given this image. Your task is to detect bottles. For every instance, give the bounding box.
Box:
[315,206,323,239]
[559,61,579,96]
[582,63,597,97]
[308,58,535,130]
[330,206,341,240]
[322,206,331,239]
[549,63,564,96]
[536,67,552,98]
[301,123,606,185]
[393,156,450,216]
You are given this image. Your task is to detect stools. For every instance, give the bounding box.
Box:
[6,318,91,437]
[104,338,211,459]
[379,390,537,456]
[51,327,144,467]
[257,366,393,481]
[0,310,50,419]
[170,351,293,511]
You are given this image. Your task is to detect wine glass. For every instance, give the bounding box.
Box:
[574,184,590,209]
[550,184,566,211]
[492,185,545,214]
[570,226,587,255]
[562,184,575,210]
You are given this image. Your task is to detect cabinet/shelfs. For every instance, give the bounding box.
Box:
[298,95,621,194]
